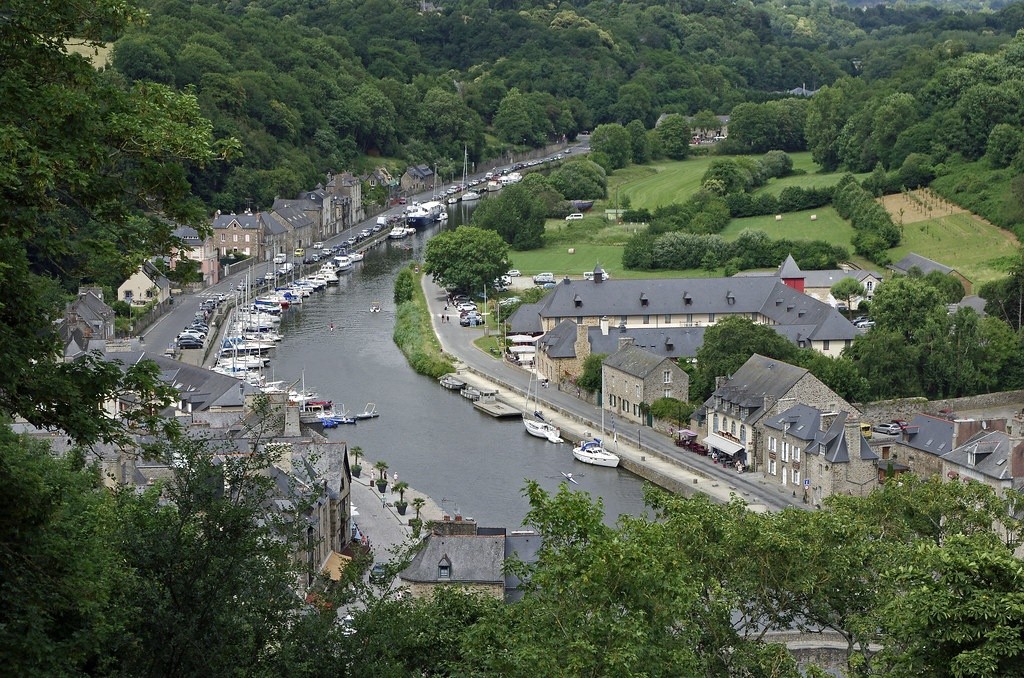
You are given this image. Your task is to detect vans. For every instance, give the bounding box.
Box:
[493,274,512,286]
[860,422,871,438]
[533,273,554,284]
[565,213,583,221]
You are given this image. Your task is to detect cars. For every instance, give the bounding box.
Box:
[453,294,485,327]
[892,420,908,430]
[368,561,390,583]
[506,269,521,276]
[537,283,555,289]
[873,422,900,435]
[293,248,305,257]
[852,315,868,325]
[493,284,509,292]
[341,612,358,638]
[313,241,325,249]
[857,319,875,328]
[447,148,572,204]
[175,300,213,349]
[273,253,286,264]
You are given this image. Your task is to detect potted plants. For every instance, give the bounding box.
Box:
[373,461,389,493]
[408,498,426,527]
[349,446,365,478]
[390,480,409,515]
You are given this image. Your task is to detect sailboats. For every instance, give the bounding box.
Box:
[521,338,564,444]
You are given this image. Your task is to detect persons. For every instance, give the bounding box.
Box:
[711,451,718,463]
[541,377,548,388]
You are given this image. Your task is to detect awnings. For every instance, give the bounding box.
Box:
[702,431,743,457]
[676,428,698,437]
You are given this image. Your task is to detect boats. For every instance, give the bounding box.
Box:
[572,440,620,467]
[213,195,447,428]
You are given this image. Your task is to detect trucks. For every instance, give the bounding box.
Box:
[583,268,610,281]
